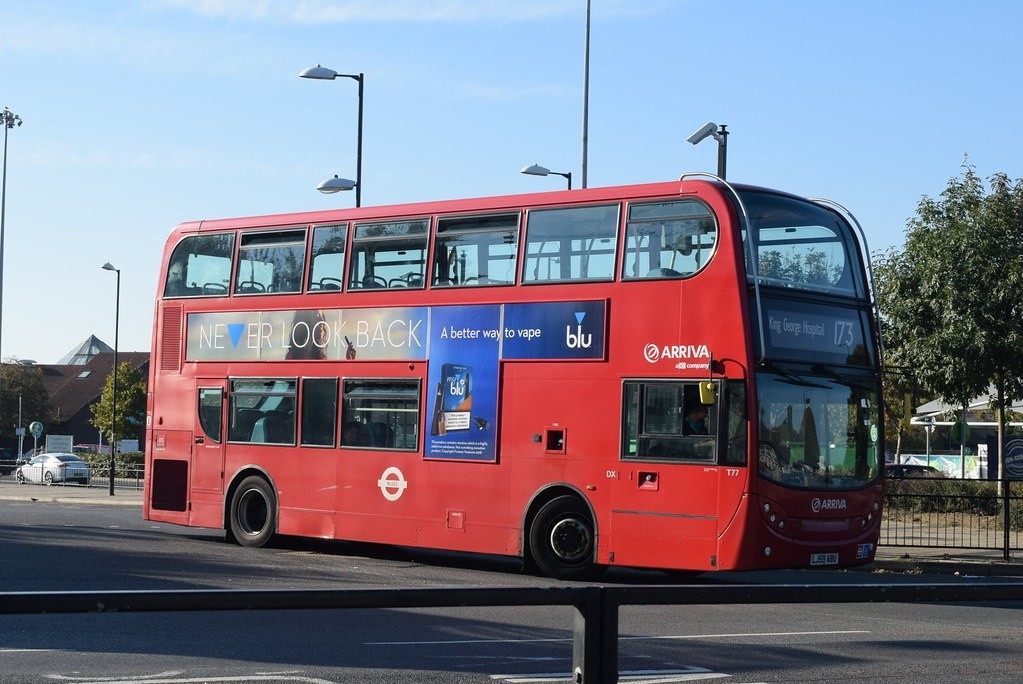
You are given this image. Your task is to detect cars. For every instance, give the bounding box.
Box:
[0,447,17,475]
[15,447,42,465]
[15,452,93,487]
[871,464,948,479]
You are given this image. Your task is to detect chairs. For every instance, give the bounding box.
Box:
[236,409,395,448]
[646,268,692,276]
[201,273,500,295]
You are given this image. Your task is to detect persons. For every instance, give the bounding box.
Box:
[682,397,708,436]
[342,421,361,447]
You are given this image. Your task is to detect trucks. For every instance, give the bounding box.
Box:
[73,444,112,454]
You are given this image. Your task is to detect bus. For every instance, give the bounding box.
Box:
[142,174,919,579]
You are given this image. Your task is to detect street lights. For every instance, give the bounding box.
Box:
[298,64,365,207]
[519,163,573,191]
[0,107,23,388]
[100,261,120,494]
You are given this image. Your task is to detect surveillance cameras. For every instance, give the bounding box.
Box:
[687,121,718,145]
